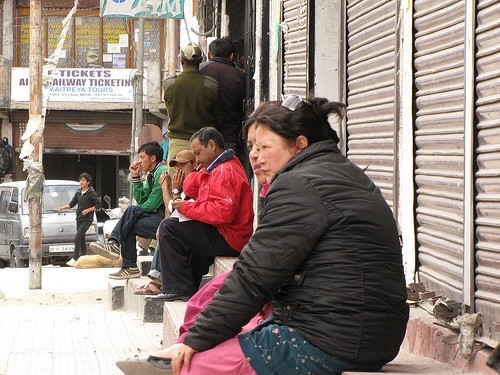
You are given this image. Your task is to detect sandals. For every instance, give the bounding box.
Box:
[134,281,163,295]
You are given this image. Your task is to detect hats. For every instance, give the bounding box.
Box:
[170,150,196,166]
[177,43,202,61]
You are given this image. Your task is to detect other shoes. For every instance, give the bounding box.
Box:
[137,250,148,256]
[115,354,174,375]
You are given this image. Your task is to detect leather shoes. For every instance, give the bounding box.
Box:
[144,292,191,302]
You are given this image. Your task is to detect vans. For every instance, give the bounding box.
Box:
[0,180,98,268]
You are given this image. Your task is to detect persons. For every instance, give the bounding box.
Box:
[242,99,283,229]
[198,36,247,160]
[115,94,410,375]
[162,42,220,216]
[59,173,102,267]
[89,140,169,280]
[133,142,239,295]
[144,126,254,302]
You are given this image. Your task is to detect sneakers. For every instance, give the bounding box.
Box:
[108,267,140,279]
[66,258,77,267]
[89,241,120,260]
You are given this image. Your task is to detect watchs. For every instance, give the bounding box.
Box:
[171,188,180,195]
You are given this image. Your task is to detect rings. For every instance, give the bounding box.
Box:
[175,351,184,358]
